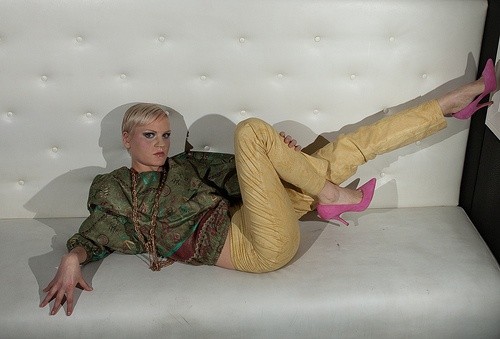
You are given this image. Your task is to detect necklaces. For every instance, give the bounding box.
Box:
[133,161,172,269]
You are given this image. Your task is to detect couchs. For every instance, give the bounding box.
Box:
[0,0,500,339]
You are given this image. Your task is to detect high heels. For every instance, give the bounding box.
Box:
[317,177,376,226]
[453,59,497,120]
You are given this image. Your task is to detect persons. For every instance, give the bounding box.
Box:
[39,59,500,317]
